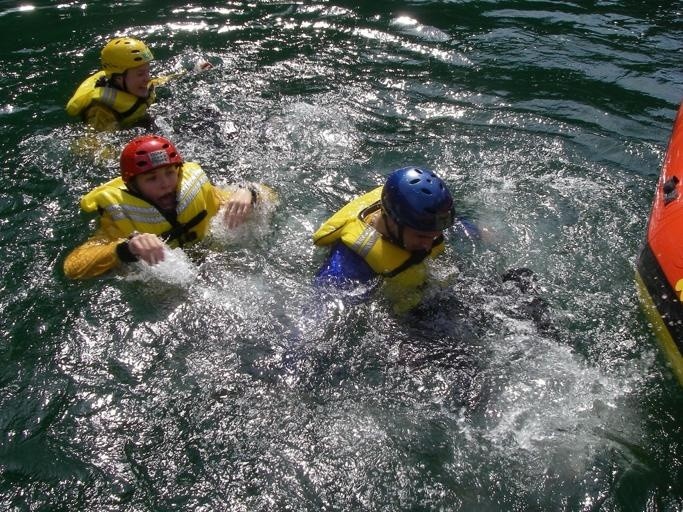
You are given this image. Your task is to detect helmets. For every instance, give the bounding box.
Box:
[381,167,455,231]
[120,135,182,182]
[101,37,154,79]
